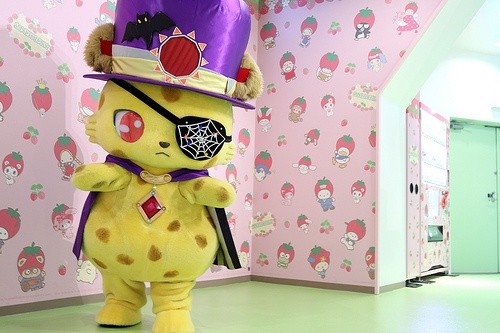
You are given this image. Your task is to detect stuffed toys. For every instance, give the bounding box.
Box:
[70,0,263,332]
